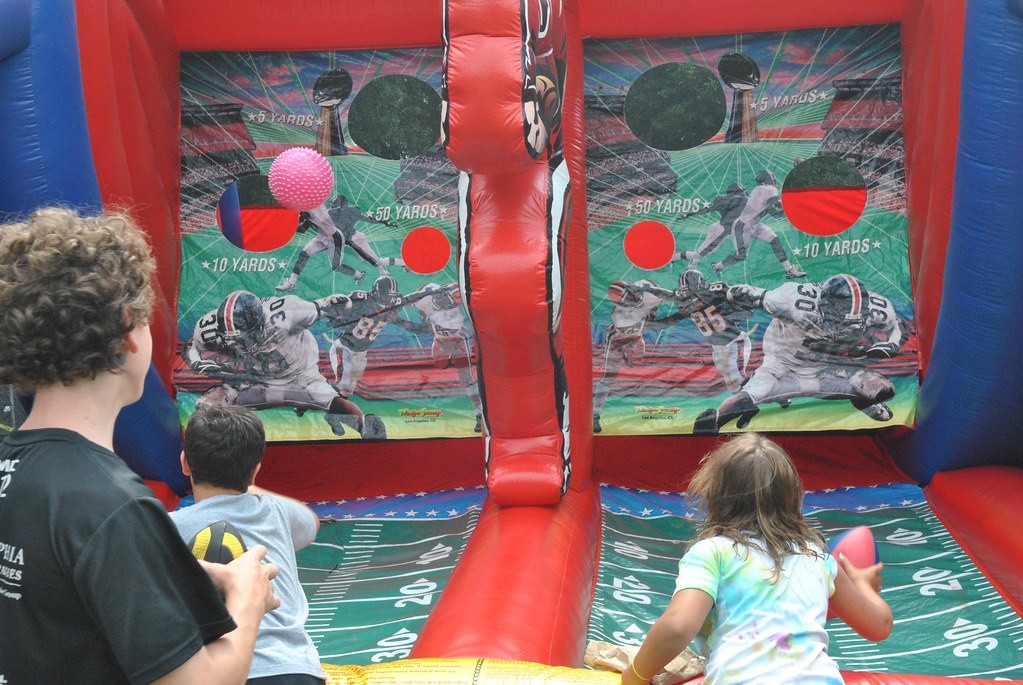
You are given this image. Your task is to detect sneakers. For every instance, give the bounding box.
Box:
[711,262,723,280]
[355,270,367,287]
[275,278,297,291]
[785,265,808,279]
[736,405,761,430]
[323,414,348,436]
[474,412,482,433]
[590,413,602,434]
[858,399,893,422]
[692,409,719,436]
[358,409,386,437]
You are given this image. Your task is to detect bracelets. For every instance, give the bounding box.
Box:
[631,660,654,682]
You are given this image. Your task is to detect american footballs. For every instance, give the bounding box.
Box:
[826,524,880,620]
[187,521,249,565]
[860,326,888,350]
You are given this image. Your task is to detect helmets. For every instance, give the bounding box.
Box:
[430,281,461,311]
[755,170,777,188]
[819,273,869,341]
[610,281,646,309]
[726,184,747,196]
[330,194,349,212]
[372,277,398,307]
[215,290,266,353]
[677,268,706,299]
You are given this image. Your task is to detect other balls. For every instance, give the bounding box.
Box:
[268,146,333,212]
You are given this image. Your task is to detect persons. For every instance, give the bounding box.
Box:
[648,269,796,413]
[667,182,755,265]
[276,273,404,437]
[0,206,280,685]
[310,192,397,278]
[692,268,911,432]
[153,396,333,684]
[272,198,365,295]
[713,171,808,283]
[179,285,388,439]
[592,274,684,434]
[383,281,487,437]
[619,430,896,684]
